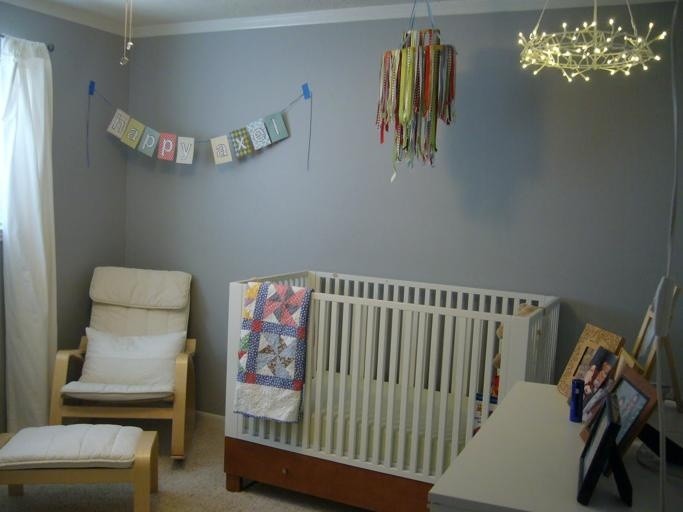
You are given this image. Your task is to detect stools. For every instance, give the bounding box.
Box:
[0,423,159,512]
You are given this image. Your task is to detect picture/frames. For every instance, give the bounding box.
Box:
[555,305,682,508]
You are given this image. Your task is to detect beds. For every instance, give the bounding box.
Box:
[220,266,564,509]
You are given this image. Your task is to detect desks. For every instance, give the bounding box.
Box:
[425,380,682,512]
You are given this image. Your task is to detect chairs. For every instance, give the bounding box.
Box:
[46,265,197,460]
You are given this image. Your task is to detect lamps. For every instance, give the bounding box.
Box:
[517,0,668,83]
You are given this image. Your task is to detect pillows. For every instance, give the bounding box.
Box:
[77,326,187,385]
